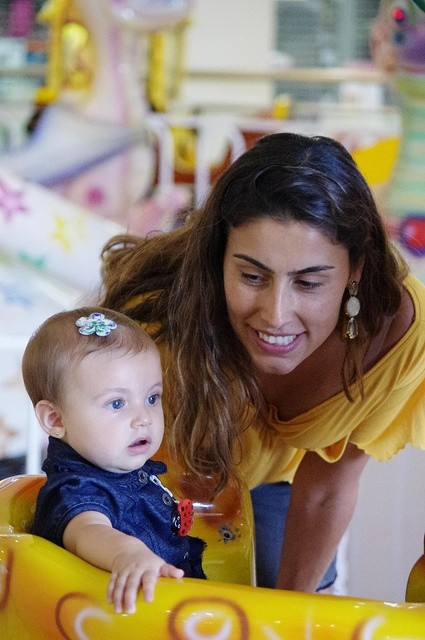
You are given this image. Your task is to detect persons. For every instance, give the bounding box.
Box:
[21,307,208,614]
[84,132,424,593]
